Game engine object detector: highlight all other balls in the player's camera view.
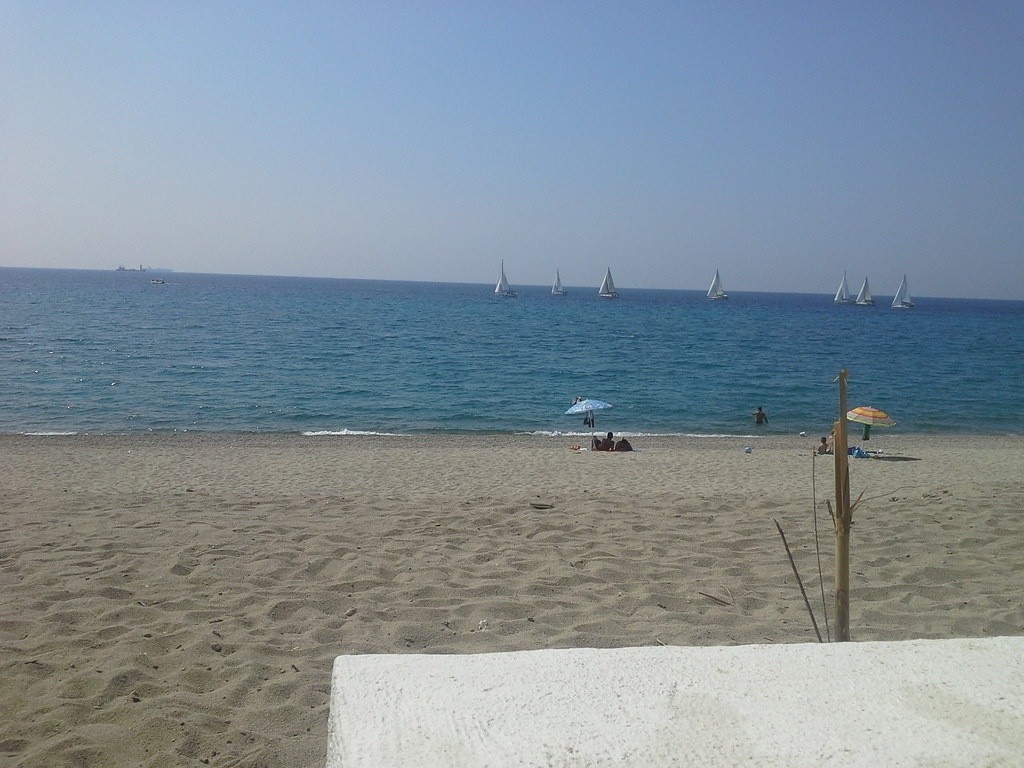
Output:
[745,447,752,453]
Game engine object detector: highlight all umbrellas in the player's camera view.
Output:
[847,406,897,451]
[565,398,612,450]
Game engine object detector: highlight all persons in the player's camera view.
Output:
[592,432,615,451]
[828,429,835,454]
[817,436,828,455]
[753,407,769,424]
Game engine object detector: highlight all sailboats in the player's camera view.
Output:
[854,276,876,305]
[833,271,855,302]
[706,268,727,299]
[552,268,565,295]
[494,259,517,297]
[598,267,618,298]
[891,274,915,309]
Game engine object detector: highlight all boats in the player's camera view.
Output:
[150,279,167,284]
